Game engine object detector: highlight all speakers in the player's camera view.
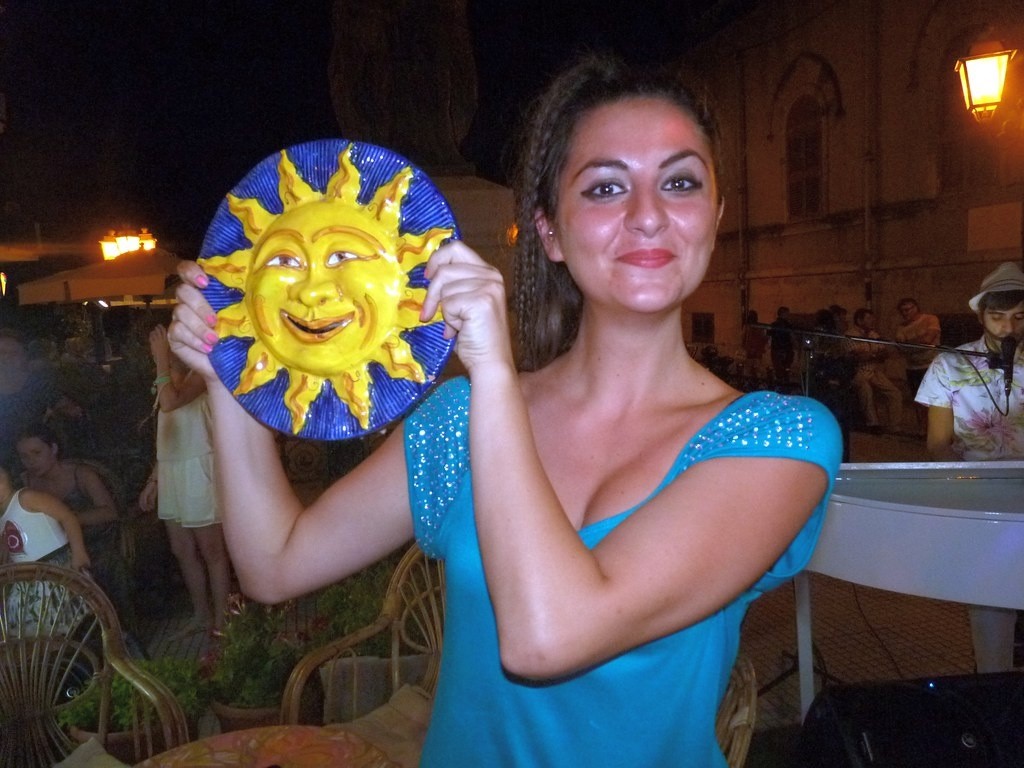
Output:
[797,669,1024,768]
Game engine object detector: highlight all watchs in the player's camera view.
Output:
[145,476,158,484]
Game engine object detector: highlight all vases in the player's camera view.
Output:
[207,684,317,733]
[320,652,429,722]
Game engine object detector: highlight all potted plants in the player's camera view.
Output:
[55,657,207,765]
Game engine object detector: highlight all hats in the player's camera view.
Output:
[968,261,1024,313]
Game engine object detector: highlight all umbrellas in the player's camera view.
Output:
[16,242,192,320]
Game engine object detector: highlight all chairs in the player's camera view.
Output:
[0,560,189,768]
[59,459,152,661]
[279,542,445,725]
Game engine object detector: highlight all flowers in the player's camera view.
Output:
[309,557,441,658]
[201,592,318,708]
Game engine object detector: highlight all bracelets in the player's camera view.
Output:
[150,367,174,395]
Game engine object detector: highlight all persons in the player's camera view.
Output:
[0,461,93,642]
[136,303,234,641]
[895,295,942,441]
[163,48,847,768]
[843,305,909,438]
[61,315,114,363]
[913,256,1024,678]
[745,303,848,398]
[11,423,136,633]
[1,326,86,494]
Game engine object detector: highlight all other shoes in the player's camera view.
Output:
[167,610,212,639]
[889,429,905,436]
[870,424,884,434]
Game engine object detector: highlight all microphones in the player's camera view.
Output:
[999,336,1017,398]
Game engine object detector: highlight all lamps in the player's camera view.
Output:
[954,24,1024,149]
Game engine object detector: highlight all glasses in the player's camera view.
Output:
[900,304,916,315]
